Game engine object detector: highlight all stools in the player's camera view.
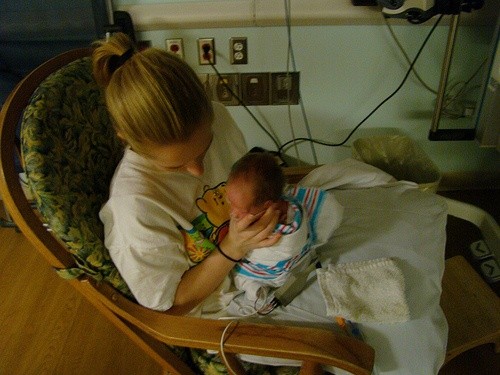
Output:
[439,255,500,370]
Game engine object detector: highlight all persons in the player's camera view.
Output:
[223,153,311,300]
[92,31,336,375]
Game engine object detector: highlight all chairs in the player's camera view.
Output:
[0,47,379,375]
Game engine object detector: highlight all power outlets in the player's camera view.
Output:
[165,39,185,60]
[197,38,216,65]
[196,71,301,107]
[229,37,247,65]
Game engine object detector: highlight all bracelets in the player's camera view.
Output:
[218,241,248,265]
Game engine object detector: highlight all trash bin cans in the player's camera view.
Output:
[353,135,442,193]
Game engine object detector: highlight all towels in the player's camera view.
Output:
[316,256,410,324]
